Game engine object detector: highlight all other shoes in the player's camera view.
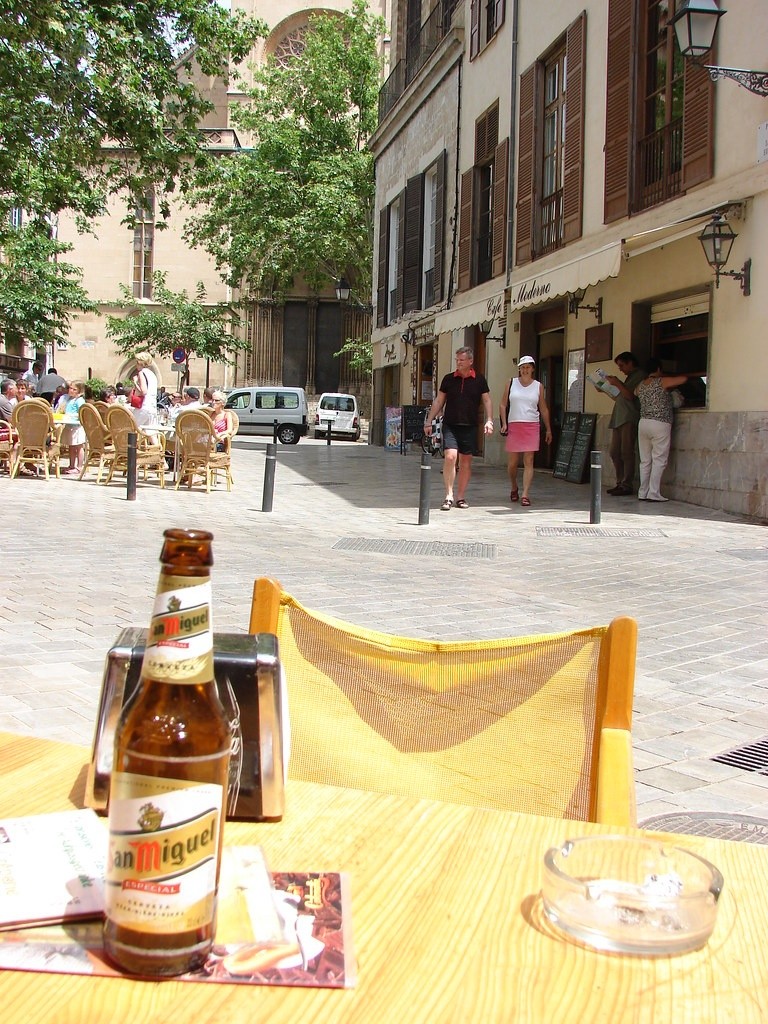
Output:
[639,495,669,503]
[24,462,42,472]
[61,465,76,474]
[66,467,82,473]
[20,468,35,476]
[606,486,633,495]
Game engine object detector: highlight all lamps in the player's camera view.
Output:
[333,277,373,317]
[399,329,415,347]
[477,319,506,349]
[568,287,602,324]
[665,0,768,98]
[697,211,751,297]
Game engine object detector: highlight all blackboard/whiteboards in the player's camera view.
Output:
[552,411,581,480]
[565,412,598,483]
[400,405,426,443]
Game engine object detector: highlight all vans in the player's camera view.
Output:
[314,392,364,441]
[222,385,310,445]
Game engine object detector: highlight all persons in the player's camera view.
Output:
[595,352,647,495]
[129,352,169,469]
[126,386,233,482]
[0,359,136,476]
[499,356,552,506]
[634,357,690,501]
[424,346,494,510]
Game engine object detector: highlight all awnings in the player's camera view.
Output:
[432,289,512,335]
[510,212,719,314]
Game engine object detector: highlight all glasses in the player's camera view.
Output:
[211,399,220,402]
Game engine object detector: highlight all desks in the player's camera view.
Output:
[0,728,768,1024]
[140,424,218,483]
[53,419,81,425]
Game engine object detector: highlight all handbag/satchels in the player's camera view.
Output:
[499,378,514,436]
[130,395,144,408]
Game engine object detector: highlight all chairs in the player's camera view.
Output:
[247,577,636,829]
[256,395,263,408]
[344,405,352,410]
[328,404,333,410]
[275,396,285,408]
[0,397,238,493]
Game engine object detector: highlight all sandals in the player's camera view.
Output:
[456,499,468,508]
[441,500,453,510]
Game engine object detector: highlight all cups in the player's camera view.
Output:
[148,409,174,426]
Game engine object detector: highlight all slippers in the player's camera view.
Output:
[521,495,531,505]
[510,486,519,502]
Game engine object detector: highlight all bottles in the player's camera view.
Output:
[102,528,232,977]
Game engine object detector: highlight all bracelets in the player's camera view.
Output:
[487,420,493,425]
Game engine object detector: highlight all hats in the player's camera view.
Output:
[184,388,200,399]
[518,356,535,366]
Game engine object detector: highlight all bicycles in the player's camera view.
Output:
[419,403,445,458]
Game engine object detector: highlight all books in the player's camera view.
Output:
[0,809,359,990]
